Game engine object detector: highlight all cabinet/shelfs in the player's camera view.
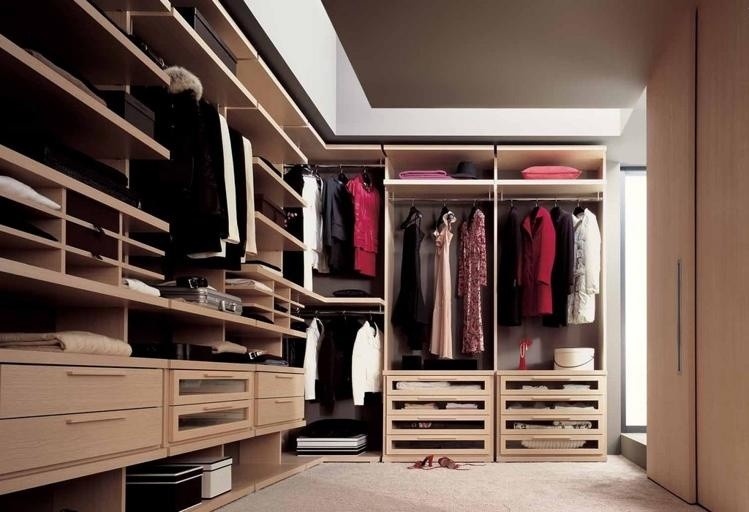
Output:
[1,2,383,512]
[384,144,607,463]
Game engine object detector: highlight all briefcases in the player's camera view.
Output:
[154,286,242,315]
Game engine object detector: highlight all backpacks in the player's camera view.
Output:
[553,347,595,370]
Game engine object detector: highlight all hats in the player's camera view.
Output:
[451,161,480,179]
